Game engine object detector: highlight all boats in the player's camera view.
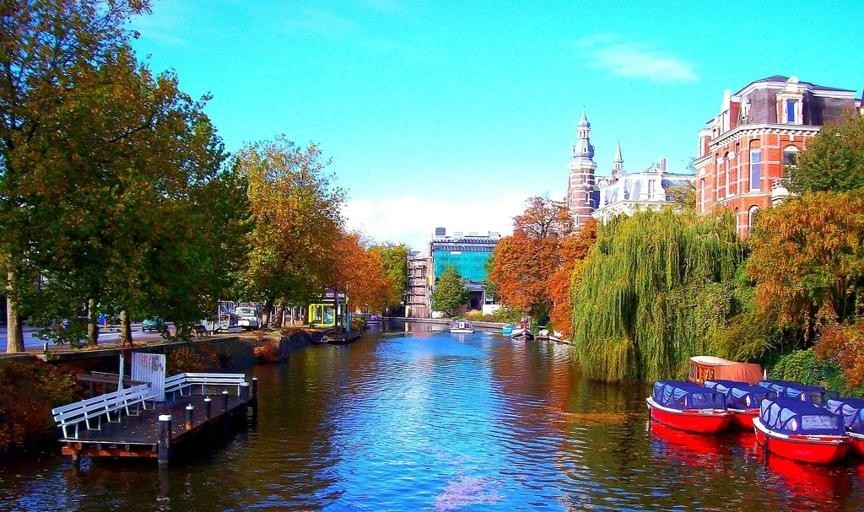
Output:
[500,321,533,339]
[646,354,864,465]
[448,319,475,333]
[306,283,362,345]
[365,314,383,324]
[645,417,863,512]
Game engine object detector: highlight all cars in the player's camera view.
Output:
[141,316,168,332]
[60,317,99,338]
